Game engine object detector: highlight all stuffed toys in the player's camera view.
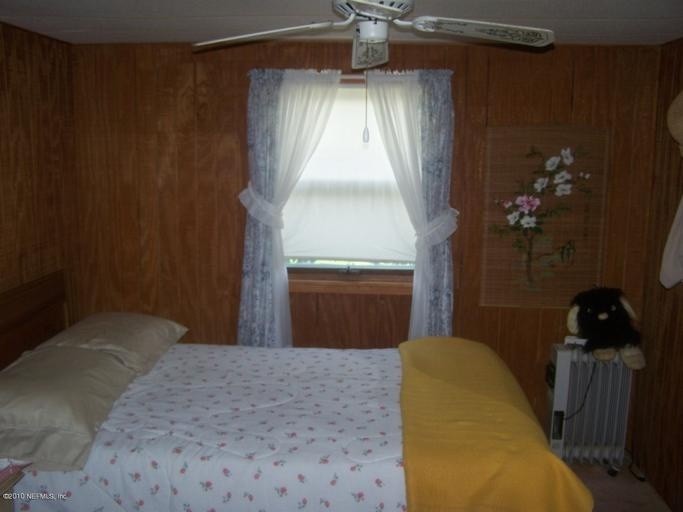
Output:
[565,286,647,370]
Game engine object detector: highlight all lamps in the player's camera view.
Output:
[357,21,388,144]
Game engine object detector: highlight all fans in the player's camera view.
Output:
[190,1,556,143]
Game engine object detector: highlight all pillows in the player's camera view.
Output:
[32,310,189,376]
[1,346,138,472]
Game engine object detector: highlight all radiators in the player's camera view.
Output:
[540,342,634,476]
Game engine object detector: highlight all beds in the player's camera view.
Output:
[0,266,593,511]
